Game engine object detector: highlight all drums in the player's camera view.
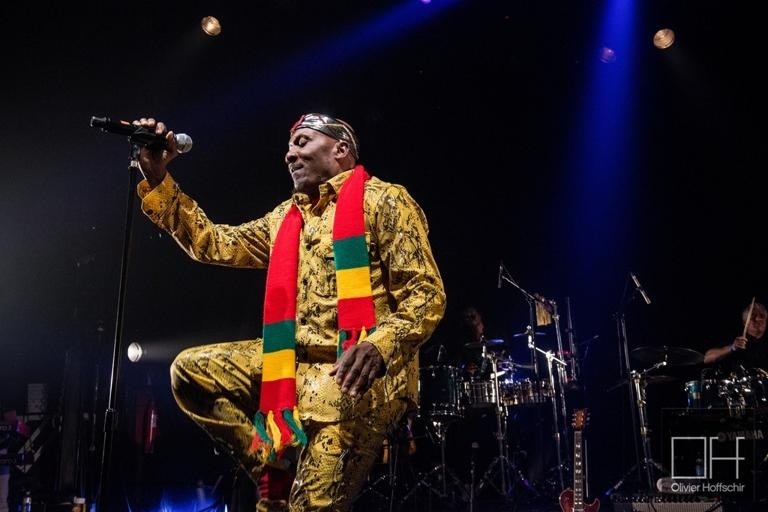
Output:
[419,366,465,421]
[500,382,524,405]
[683,380,718,410]
[546,350,580,384]
[522,378,552,404]
[470,380,497,408]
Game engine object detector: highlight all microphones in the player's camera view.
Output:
[628,270,652,305]
[88,113,195,156]
[495,258,509,288]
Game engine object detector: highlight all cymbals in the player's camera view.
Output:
[514,332,545,337]
[630,347,705,367]
[465,339,504,348]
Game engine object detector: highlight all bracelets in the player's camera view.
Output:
[729,343,735,353]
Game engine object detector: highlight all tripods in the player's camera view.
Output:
[346,302,718,511]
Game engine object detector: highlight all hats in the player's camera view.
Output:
[289,111,361,162]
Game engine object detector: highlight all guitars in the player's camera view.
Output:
[559,408,600,512]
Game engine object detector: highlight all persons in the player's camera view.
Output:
[456,305,499,373]
[132,112,448,512]
[702,302,766,371]
